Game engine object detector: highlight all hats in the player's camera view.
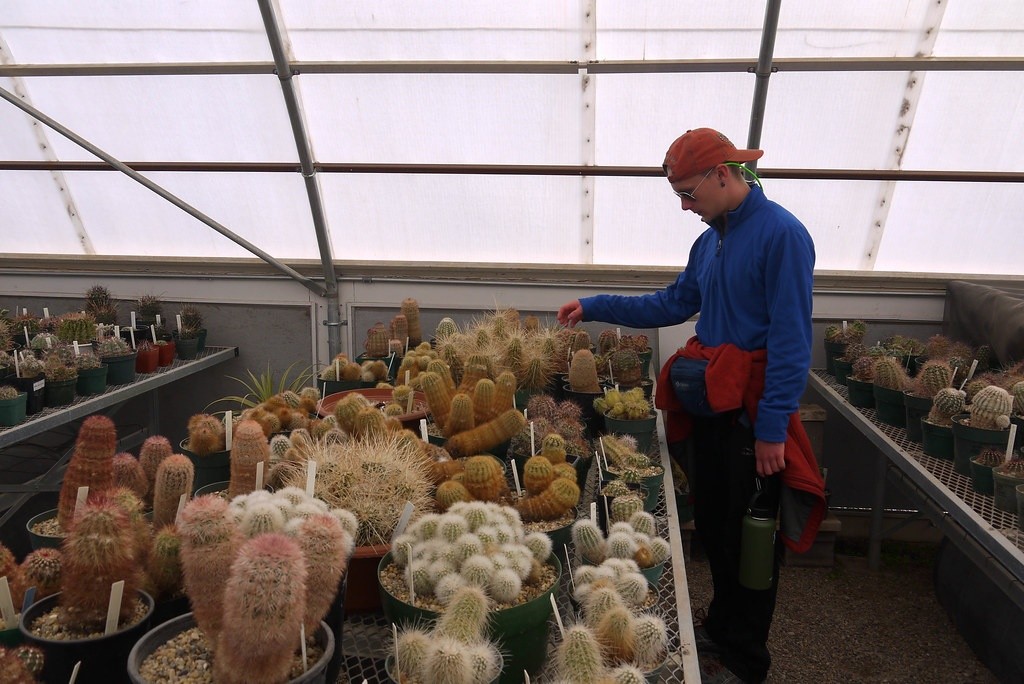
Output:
[661,127,764,183]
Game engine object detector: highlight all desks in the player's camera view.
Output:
[809,365,1024,610]
[0,346,239,525]
[0,361,706,684]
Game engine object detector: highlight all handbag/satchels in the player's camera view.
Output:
[668,353,713,417]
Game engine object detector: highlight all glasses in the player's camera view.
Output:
[673,169,714,203]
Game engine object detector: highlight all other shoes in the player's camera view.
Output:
[702,659,744,683]
[696,610,726,654]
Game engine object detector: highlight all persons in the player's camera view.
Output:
[557,132,826,684]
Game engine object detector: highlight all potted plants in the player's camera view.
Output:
[0,284,1024,684]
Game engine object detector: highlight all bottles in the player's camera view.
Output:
[738,497,777,590]
